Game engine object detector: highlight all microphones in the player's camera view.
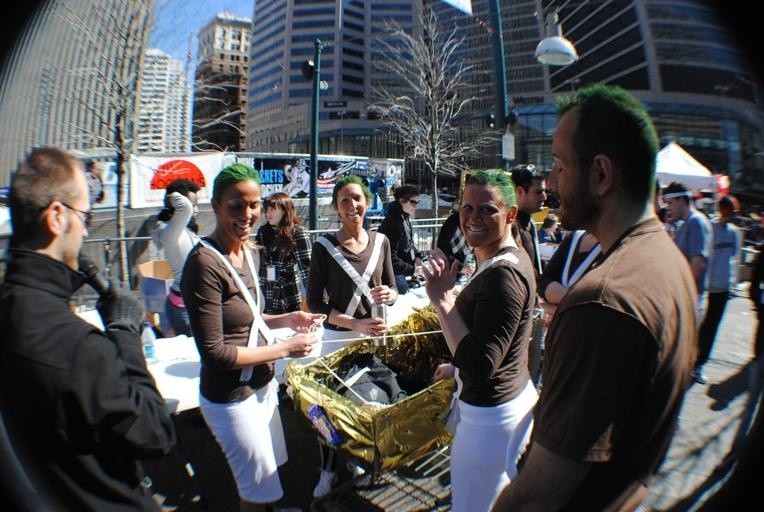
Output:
[77,254,110,297]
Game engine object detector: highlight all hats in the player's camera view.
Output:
[542,213,558,229]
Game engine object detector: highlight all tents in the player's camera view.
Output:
[655,140,714,191]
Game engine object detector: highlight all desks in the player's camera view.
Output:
[144,282,455,448]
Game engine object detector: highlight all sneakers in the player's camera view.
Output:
[693,367,708,385]
[313,470,335,498]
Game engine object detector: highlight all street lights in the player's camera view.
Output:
[484,0,580,175]
[301,36,327,236]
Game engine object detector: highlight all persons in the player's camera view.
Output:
[509,162,547,385]
[491,85,700,512]
[376,186,422,294]
[692,196,739,384]
[88,159,104,204]
[305,175,399,497]
[0,145,177,512]
[437,212,474,282]
[535,229,605,325]
[537,213,562,243]
[179,162,328,512]
[662,182,713,317]
[419,168,539,512]
[155,178,201,338]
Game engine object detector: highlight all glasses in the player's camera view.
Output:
[64,203,95,228]
[408,198,421,206]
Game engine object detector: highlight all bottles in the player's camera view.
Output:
[141,322,158,364]
[372,303,386,346]
